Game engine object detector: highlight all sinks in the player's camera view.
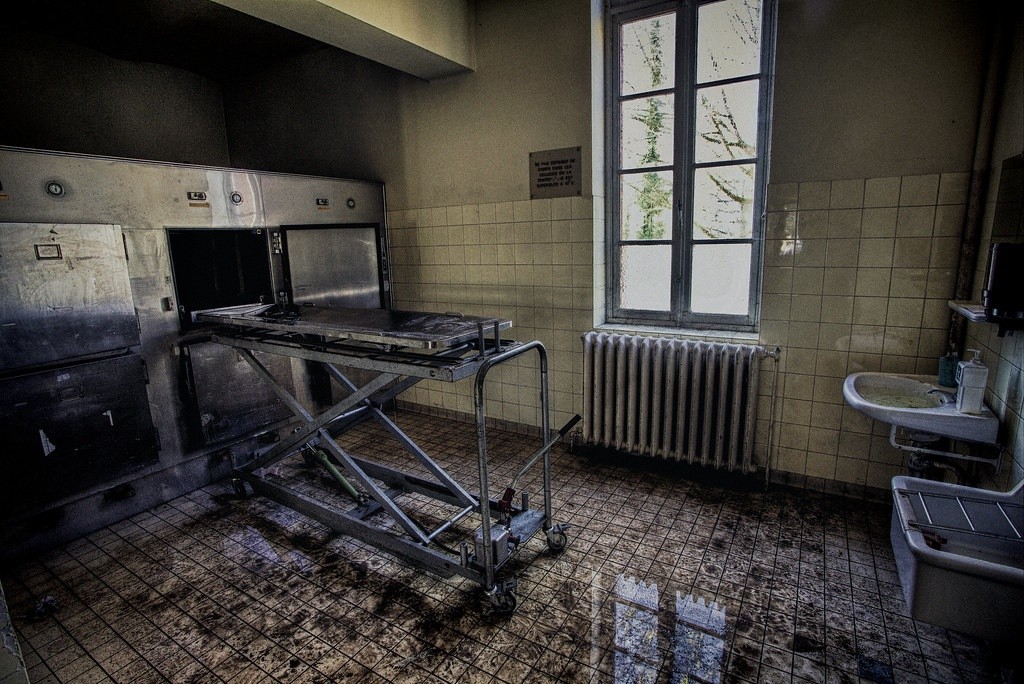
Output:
[842,371,1000,445]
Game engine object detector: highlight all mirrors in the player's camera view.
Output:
[983,153,1024,312]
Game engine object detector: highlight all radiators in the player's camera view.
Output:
[581,331,767,477]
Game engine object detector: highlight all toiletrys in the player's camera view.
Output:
[939,345,959,388]
[955,348,989,416]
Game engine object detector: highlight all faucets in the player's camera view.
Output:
[926,388,955,404]
